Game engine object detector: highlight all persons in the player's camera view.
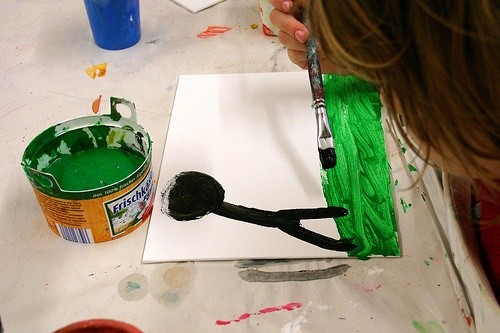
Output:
[269,0,499,299]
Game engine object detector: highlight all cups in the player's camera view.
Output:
[52,318,144,333]
[83,0,141,51]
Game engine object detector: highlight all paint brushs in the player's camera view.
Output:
[295,1,339,169]
